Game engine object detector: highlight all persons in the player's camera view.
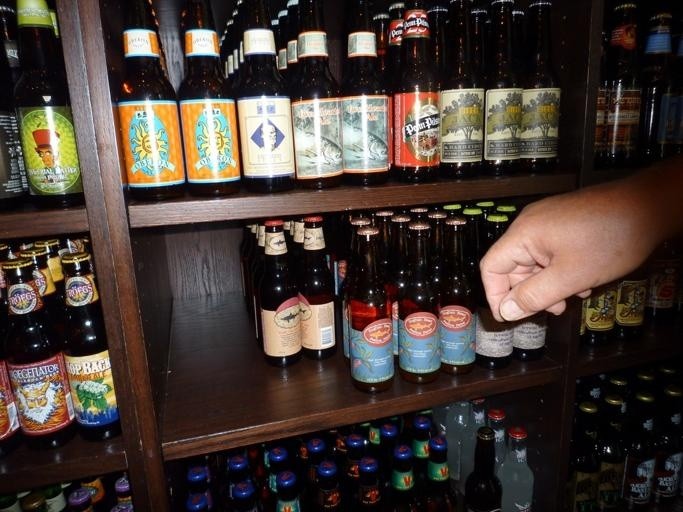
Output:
[260,125,279,152]
[480,148,683,323]
[32,129,66,184]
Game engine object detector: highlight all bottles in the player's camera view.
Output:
[1,469,132,510]
[179,380,535,510]
[1,1,86,211]
[590,3,683,173]
[565,363,681,509]
[1,230,126,454]
[579,233,681,348]
[239,194,550,399]
[99,2,588,206]
